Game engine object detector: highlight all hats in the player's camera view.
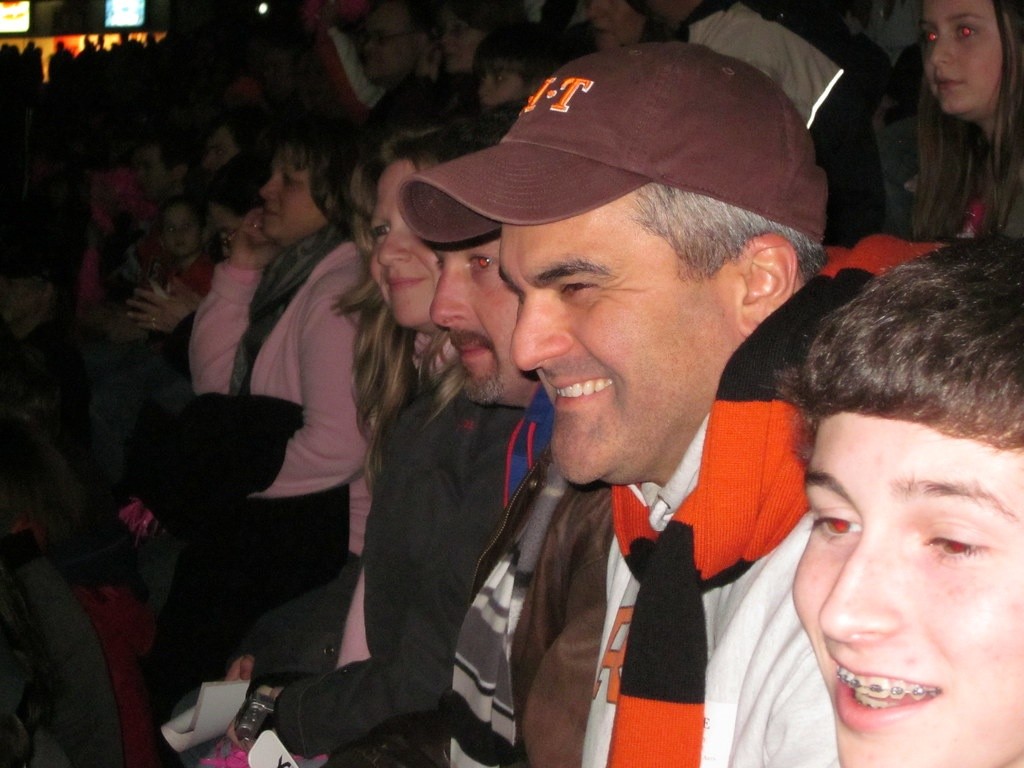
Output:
[395,41,828,242]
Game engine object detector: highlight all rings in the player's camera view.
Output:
[153,322,155,329]
[152,316,155,322]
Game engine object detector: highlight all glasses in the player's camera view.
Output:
[357,27,426,46]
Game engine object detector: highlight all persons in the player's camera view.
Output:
[793,238,1024,768]
[0,100,554,768]
[0,32,156,87]
[262,0,1024,248]
[396,41,877,768]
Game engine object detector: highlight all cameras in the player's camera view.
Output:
[235,699,276,740]
